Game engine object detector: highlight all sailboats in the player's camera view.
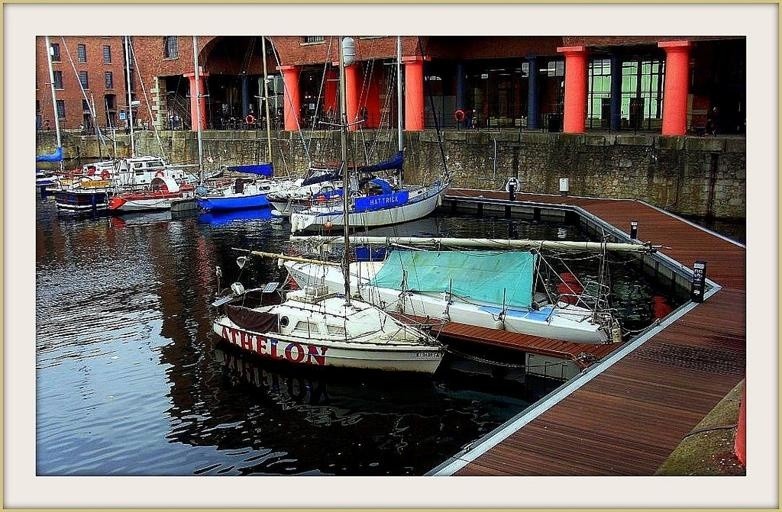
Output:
[207,43,447,373]
[229,232,667,343]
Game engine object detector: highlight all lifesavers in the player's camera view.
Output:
[455,109,465,122]
[506,177,520,192]
[246,114,255,124]
[155,170,164,178]
